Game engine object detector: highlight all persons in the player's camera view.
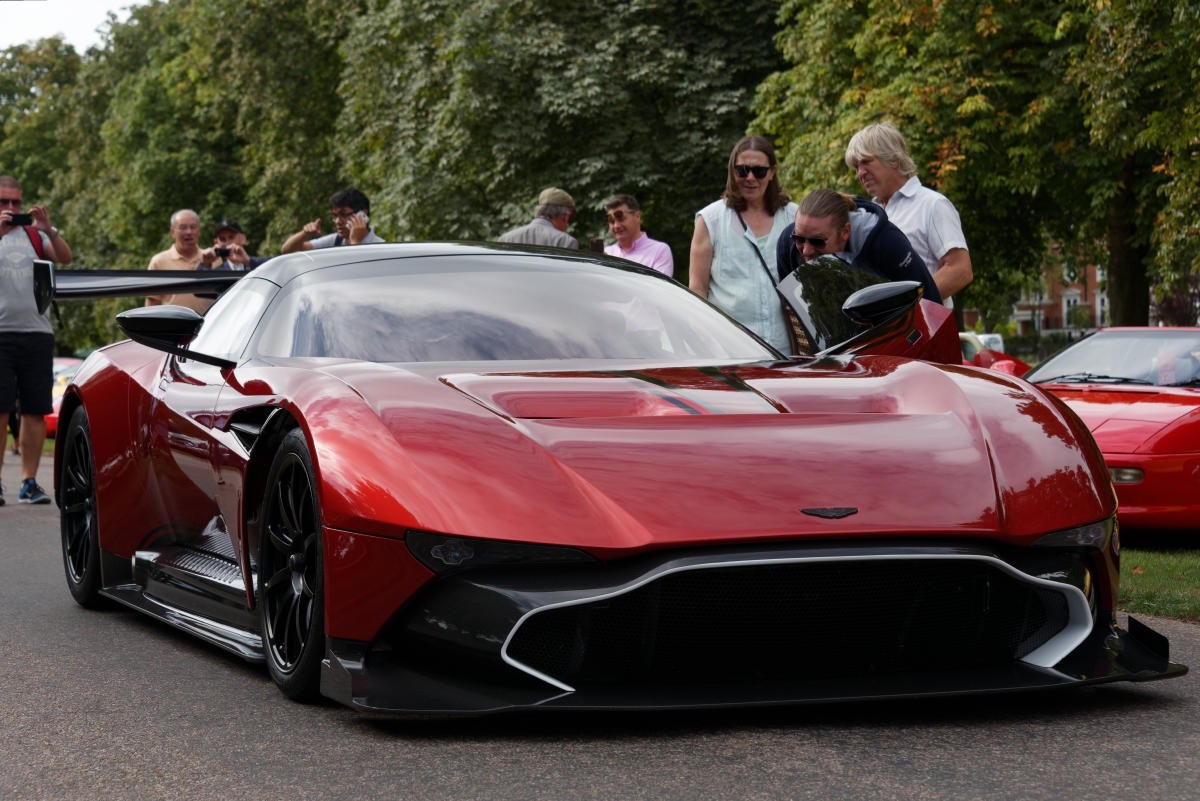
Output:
[144,209,223,316]
[281,187,386,254]
[206,433,226,485]
[7,409,20,455]
[497,187,578,250]
[776,189,944,352]
[0,175,73,507]
[599,194,673,278]
[192,219,272,300]
[844,121,973,308]
[688,136,799,359]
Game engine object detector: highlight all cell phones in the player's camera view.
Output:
[345,211,370,231]
[214,248,230,258]
[6,213,33,227]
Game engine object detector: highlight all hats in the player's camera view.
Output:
[214,220,242,237]
[539,188,576,215]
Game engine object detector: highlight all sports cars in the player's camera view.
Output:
[28,237,1191,722]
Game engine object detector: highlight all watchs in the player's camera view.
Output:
[49,226,58,239]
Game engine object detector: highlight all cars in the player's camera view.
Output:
[990,325,1200,535]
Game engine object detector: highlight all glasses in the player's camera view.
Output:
[0,198,21,207]
[330,207,361,222]
[735,163,772,180]
[789,229,840,249]
[604,208,637,224]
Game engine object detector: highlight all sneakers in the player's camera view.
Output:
[0,485,6,506]
[18,478,51,504]
